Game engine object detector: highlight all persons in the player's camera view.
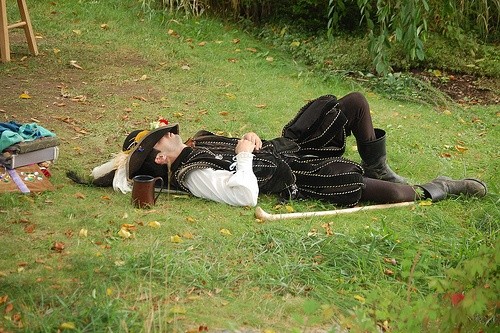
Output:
[93,92,489,205]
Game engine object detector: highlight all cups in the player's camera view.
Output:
[131,175,164,208]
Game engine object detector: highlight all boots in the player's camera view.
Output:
[416,176,488,202]
[356,127,407,184]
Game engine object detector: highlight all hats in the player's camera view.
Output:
[66,119,179,193]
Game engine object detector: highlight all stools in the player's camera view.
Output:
[0,0,39,62]
